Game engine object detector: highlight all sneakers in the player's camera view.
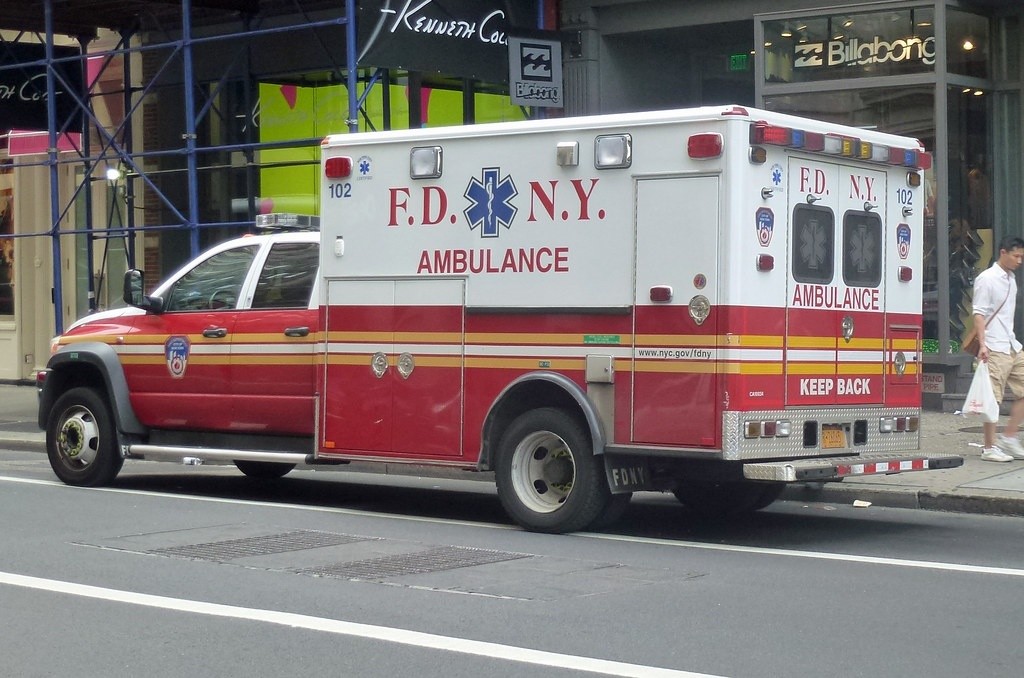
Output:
[997,436,1024,459]
[981,445,1013,462]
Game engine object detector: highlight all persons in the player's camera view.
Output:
[972,235,1024,462]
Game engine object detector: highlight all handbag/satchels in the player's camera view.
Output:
[962,360,999,423]
[960,328,980,357]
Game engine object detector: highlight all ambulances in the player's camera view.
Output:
[33,102,964,536]
